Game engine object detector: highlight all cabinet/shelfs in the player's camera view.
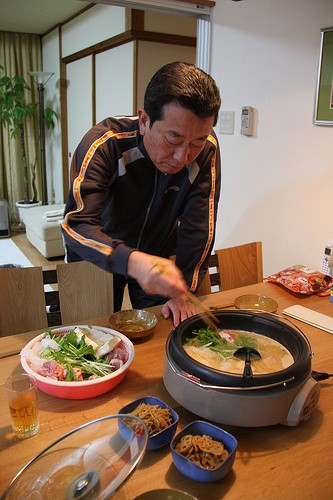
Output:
[37,5,196,205]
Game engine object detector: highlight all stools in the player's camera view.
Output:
[23,203,66,259]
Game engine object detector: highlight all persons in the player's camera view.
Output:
[61,60,222,328]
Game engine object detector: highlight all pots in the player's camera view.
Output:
[162,309,322,427]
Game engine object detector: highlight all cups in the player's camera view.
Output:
[6,373,40,439]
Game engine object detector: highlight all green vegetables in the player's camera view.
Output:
[40,328,116,382]
[188,326,262,362]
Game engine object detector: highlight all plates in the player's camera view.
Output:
[268,263,333,295]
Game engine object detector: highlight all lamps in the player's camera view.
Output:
[28,70,54,205]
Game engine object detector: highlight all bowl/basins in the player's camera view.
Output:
[108,308,158,339]
[133,488,197,500]
[20,324,135,399]
[170,420,238,484]
[118,396,179,451]
[234,294,278,313]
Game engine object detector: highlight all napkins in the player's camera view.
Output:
[282,305,333,335]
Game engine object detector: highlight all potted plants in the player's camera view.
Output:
[0,62,59,226]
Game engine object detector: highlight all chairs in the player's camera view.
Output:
[0,261,115,338]
[169,241,264,296]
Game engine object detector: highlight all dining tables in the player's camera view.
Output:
[0,281,333,500]
